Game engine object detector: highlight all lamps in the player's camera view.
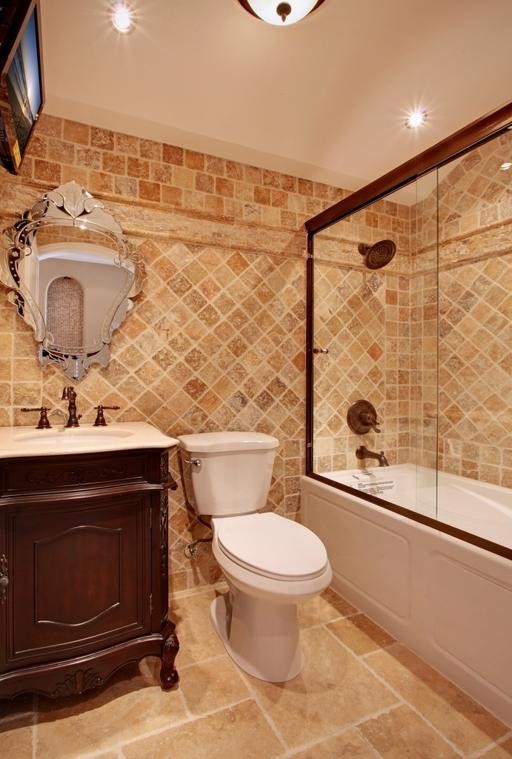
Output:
[235,0,324,25]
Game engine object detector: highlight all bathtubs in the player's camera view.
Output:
[319,461,512,550]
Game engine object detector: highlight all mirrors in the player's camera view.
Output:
[0,178,148,378]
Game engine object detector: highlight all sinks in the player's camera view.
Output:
[13,426,138,454]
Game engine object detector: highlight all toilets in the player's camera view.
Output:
[176,431,333,683]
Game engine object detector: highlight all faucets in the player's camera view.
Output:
[61,385,82,428]
[356,444,389,467]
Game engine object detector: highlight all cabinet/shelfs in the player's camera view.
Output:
[1,451,179,707]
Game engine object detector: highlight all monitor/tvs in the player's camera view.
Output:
[0,1,46,176]
